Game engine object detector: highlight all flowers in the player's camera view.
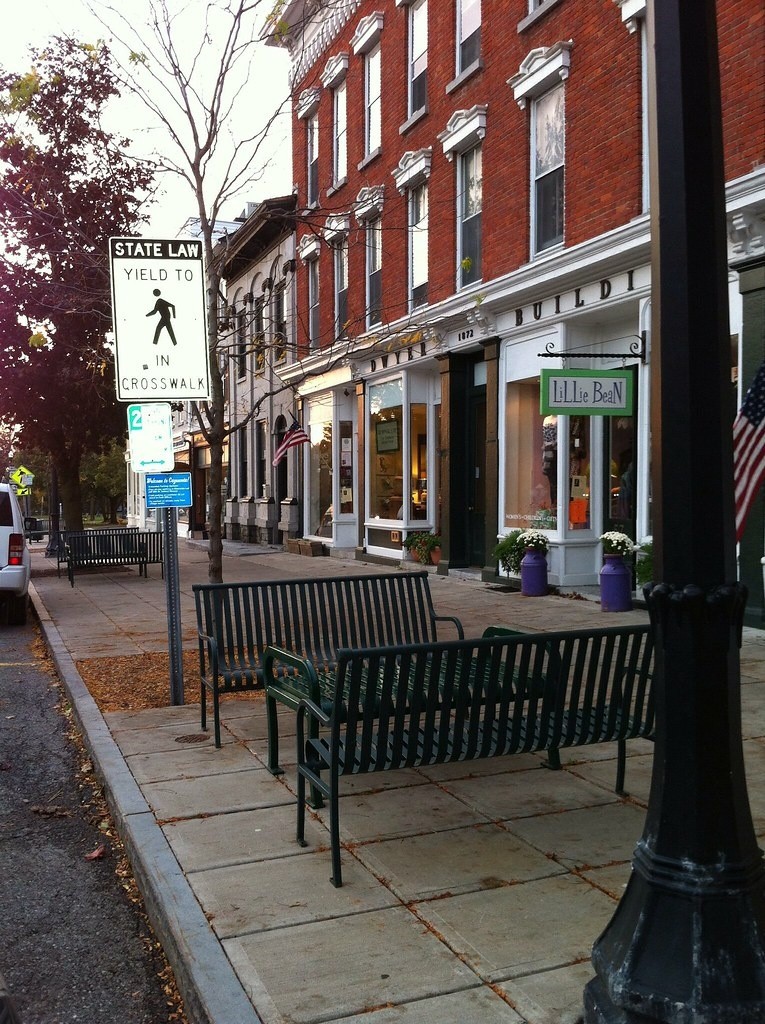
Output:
[634,535,654,591]
[599,531,634,555]
[516,531,551,555]
[399,530,441,565]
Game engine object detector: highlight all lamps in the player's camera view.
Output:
[416,478,427,502]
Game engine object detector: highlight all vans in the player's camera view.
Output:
[0,482,37,626]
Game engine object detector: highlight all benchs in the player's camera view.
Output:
[68,542,148,582]
[191,570,464,749]
[67,531,165,588]
[262,624,561,811]
[26,519,65,544]
[57,527,143,579]
[295,623,656,889]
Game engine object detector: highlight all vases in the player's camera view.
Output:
[604,554,621,559]
[524,547,538,553]
[410,546,421,562]
[298,540,322,557]
[428,546,441,565]
[286,537,309,554]
